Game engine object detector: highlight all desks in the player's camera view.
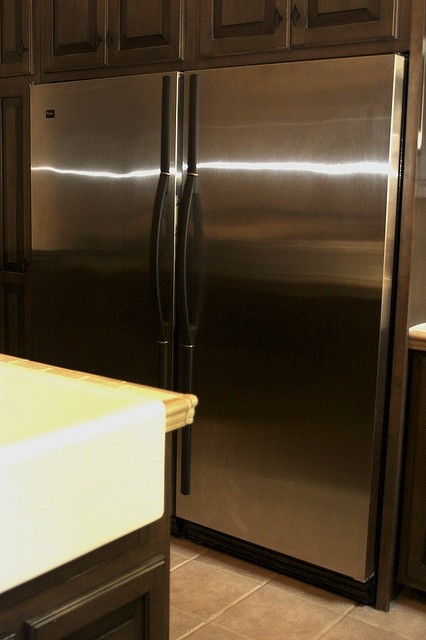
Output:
[0,354,198,640]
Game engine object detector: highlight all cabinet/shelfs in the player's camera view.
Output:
[0,0,37,77]
[37,0,193,72]
[0,79,29,275]
[1,273,27,360]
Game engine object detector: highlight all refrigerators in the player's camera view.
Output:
[30,51,410,582]
